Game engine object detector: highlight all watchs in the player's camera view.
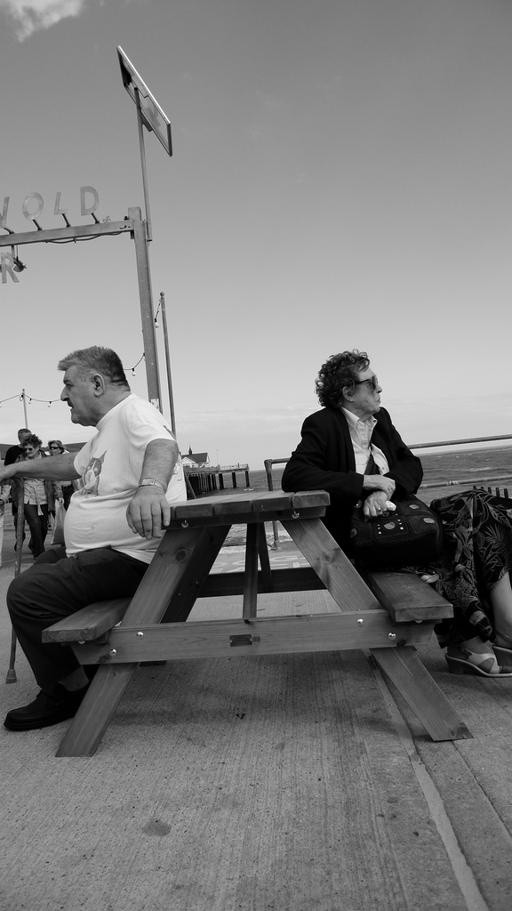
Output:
[137,478,163,488]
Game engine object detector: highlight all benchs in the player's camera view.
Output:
[42,590,164,760]
[366,563,475,743]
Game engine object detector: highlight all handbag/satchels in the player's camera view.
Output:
[341,499,439,569]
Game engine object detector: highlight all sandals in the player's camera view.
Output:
[446,630,512,677]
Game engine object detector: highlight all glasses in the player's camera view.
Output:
[357,376,377,390]
[50,447,58,449]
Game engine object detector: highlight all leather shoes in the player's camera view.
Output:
[2,690,84,731]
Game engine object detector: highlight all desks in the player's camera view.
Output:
[58,487,477,754]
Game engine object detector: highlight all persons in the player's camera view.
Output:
[282,351,512,677]
[0,345,186,731]
[0,428,75,558]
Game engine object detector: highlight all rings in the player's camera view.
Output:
[376,508,382,512]
